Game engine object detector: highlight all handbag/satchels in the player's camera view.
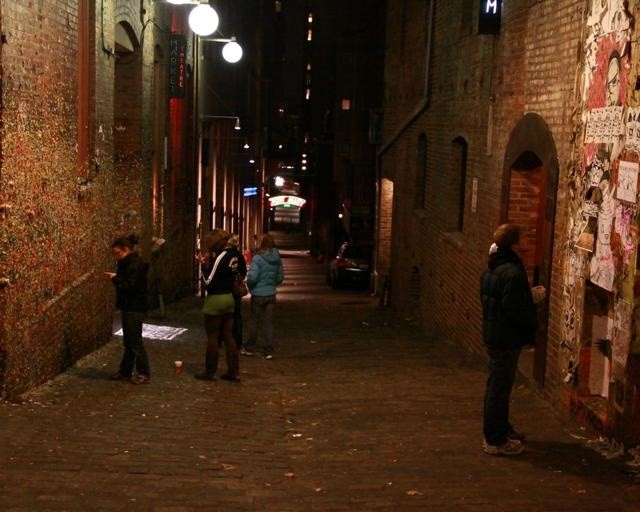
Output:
[231,277,248,299]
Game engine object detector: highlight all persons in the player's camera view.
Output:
[103,234,154,384]
[479,222,546,456]
[196,228,284,383]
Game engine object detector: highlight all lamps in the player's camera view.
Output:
[206,114,241,131]
[200,35,245,63]
[154,0,219,36]
[224,134,251,150]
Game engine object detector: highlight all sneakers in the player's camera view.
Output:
[220,372,241,382]
[260,353,274,359]
[194,369,217,381]
[483,438,522,457]
[105,372,133,382]
[130,374,154,384]
[240,348,256,357]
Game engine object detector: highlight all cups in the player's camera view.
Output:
[174,360,182,374]
